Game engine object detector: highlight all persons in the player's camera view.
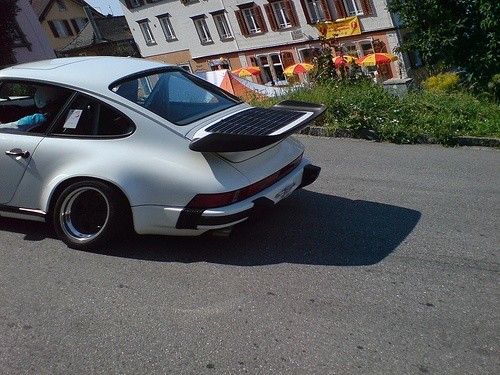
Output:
[377,65,383,82]
[0,85,59,130]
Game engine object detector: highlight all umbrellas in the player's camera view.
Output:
[283,63,315,77]
[355,52,398,81]
[327,55,358,68]
[231,66,262,79]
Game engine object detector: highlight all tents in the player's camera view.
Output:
[194,69,297,106]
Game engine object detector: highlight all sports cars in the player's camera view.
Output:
[0,55,328,252]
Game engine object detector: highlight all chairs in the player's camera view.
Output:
[64,102,100,139]
